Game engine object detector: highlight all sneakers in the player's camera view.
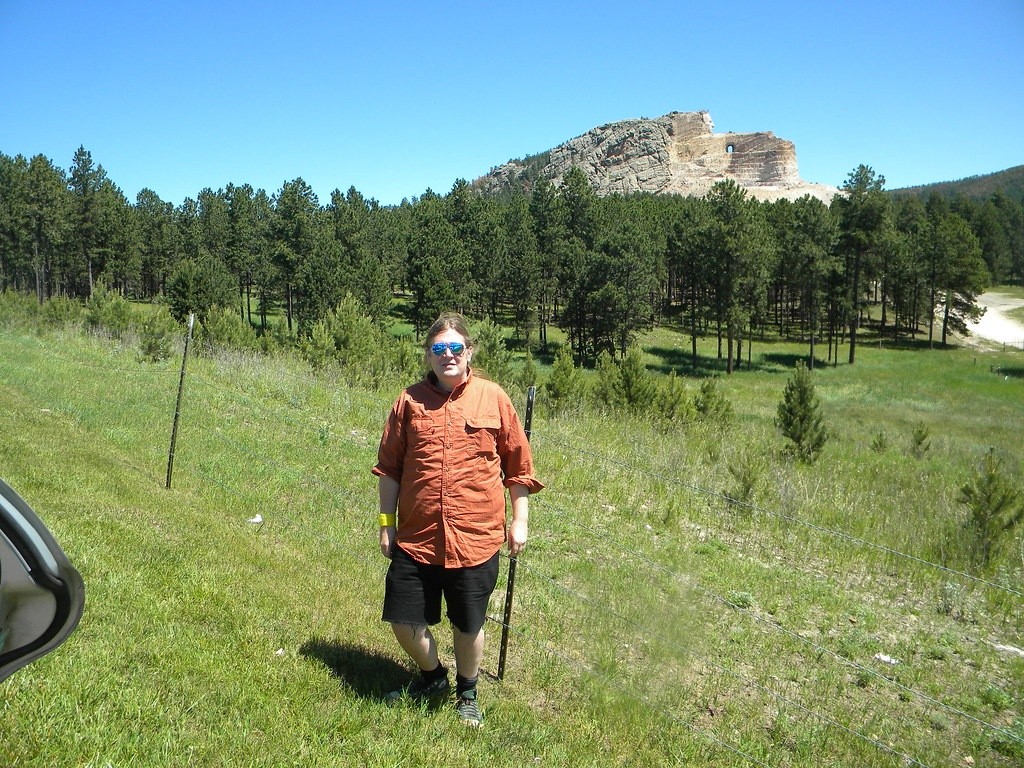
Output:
[389,671,449,702]
[450,683,480,728]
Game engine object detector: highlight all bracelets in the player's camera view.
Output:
[377,514,396,528]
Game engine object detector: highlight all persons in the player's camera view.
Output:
[369,313,546,728]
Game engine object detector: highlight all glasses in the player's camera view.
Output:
[428,342,468,356]
